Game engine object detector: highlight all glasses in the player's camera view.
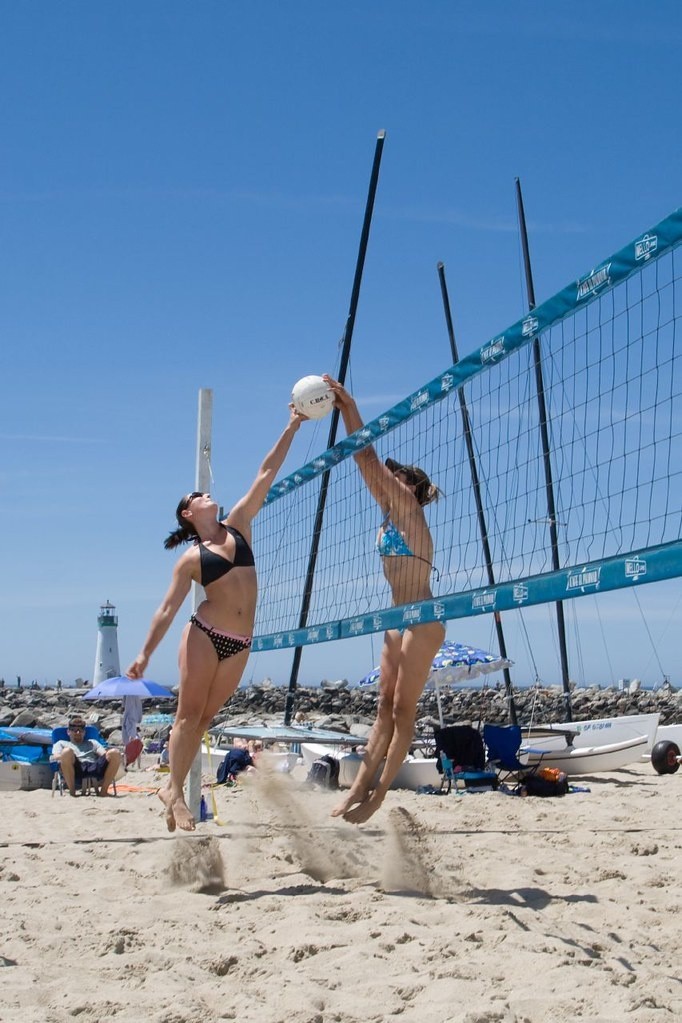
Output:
[68,725,86,732]
[184,492,202,510]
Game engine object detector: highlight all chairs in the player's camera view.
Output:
[51,726,117,797]
[433,725,498,794]
[484,725,552,796]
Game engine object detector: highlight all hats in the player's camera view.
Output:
[386,457,420,496]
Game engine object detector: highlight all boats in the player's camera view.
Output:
[291,710,661,789]
[638,724,682,762]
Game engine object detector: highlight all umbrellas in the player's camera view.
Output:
[142,712,174,740]
[81,677,177,701]
[358,639,515,728]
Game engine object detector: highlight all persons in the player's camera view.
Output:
[126,402,309,831]
[129,726,142,769]
[141,734,152,747]
[51,718,122,797]
[322,372,445,825]
[161,741,170,764]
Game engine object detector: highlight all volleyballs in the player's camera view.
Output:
[292,377,334,418]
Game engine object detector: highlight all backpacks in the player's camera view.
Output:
[306,755,339,790]
